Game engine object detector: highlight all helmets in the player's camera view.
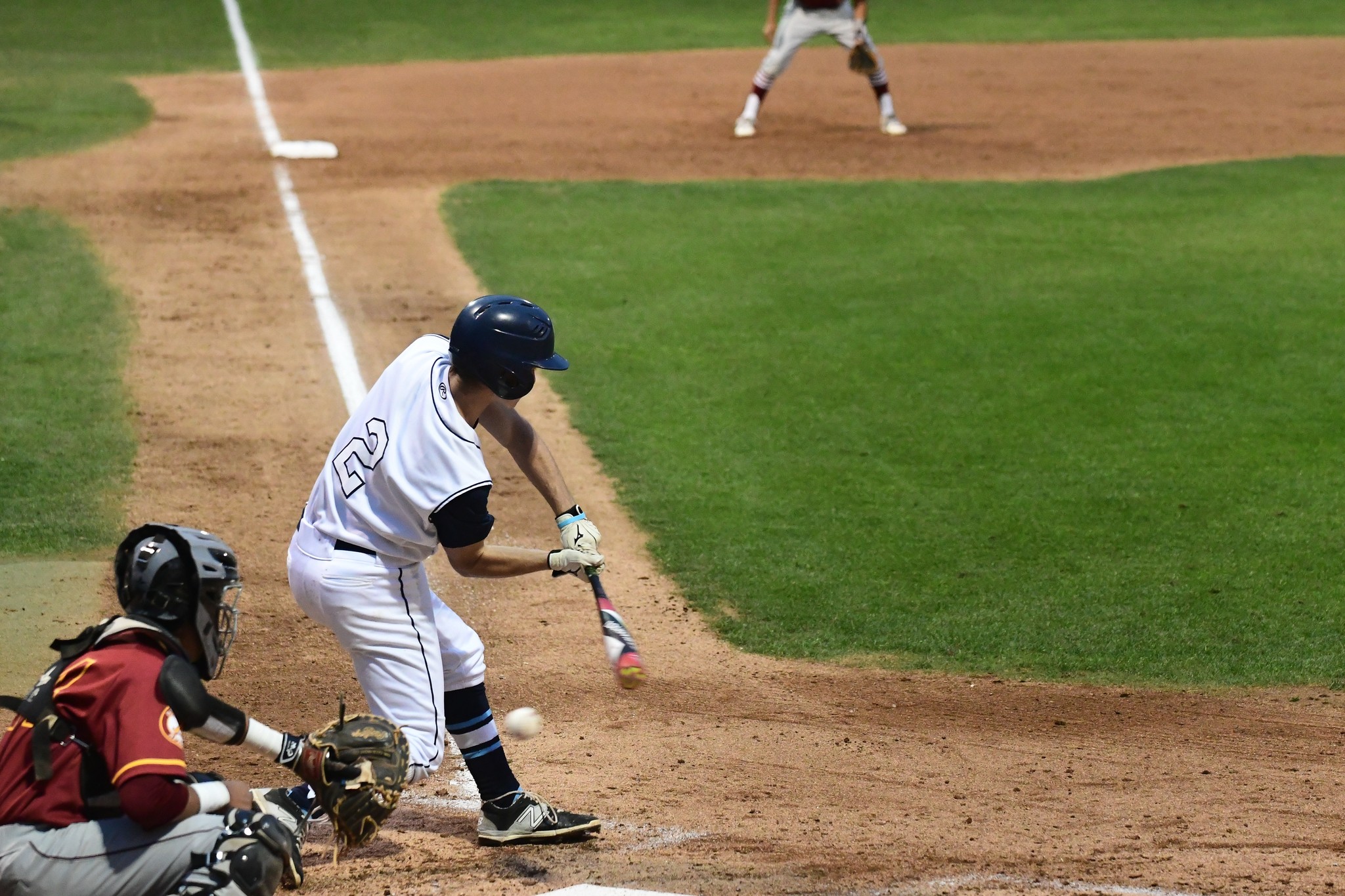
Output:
[449,296,569,400]
[113,521,241,681]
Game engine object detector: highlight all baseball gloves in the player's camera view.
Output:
[301,713,407,848]
[849,39,878,75]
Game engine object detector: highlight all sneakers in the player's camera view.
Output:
[733,115,755,138]
[477,787,602,845]
[248,788,329,854]
[881,118,906,137]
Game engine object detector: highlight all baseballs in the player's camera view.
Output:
[500,708,547,739]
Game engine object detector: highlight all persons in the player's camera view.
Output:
[733,0,908,137]
[286,294,606,847]
[0,522,362,896]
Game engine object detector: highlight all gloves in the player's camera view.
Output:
[547,545,606,583]
[555,503,601,551]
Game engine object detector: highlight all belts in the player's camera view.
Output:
[296,508,376,556]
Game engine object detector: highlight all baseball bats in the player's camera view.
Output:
[585,563,647,690]
